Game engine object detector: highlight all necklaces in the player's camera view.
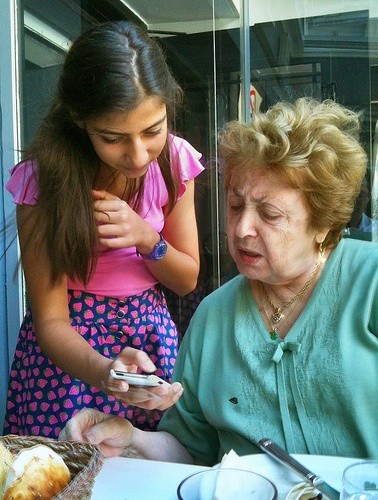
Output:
[257,248,327,339]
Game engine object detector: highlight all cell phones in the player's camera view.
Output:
[110,369,171,388]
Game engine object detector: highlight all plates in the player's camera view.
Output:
[199,452,377,499]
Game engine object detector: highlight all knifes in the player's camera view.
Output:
[258,437,340,500]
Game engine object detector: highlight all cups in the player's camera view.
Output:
[176,468,278,500]
[340,460,378,500]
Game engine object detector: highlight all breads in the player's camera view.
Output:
[0,441,71,500]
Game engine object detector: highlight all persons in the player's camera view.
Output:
[58,98,378,466]
[3,22,204,442]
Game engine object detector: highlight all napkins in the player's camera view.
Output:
[213,449,314,500]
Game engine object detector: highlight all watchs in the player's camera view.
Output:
[136,232,168,261]
